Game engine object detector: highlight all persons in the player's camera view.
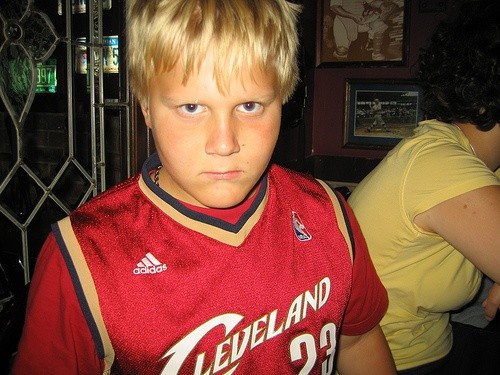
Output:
[15,0,398,375]
[330,0,392,61]
[347,0,500,375]
[358,97,417,132]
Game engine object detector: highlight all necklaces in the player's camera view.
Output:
[153,162,163,187]
[451,123,478,158]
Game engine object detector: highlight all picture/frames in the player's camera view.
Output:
[343,78,426,149]
[317,0,410,69]
